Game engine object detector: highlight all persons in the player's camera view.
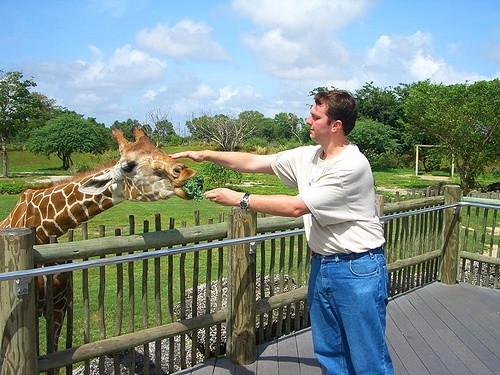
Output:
[169,90,398,375]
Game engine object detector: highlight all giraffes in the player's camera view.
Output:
[0,124,199,375]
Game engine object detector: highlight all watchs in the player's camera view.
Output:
[239,194,250,210]
